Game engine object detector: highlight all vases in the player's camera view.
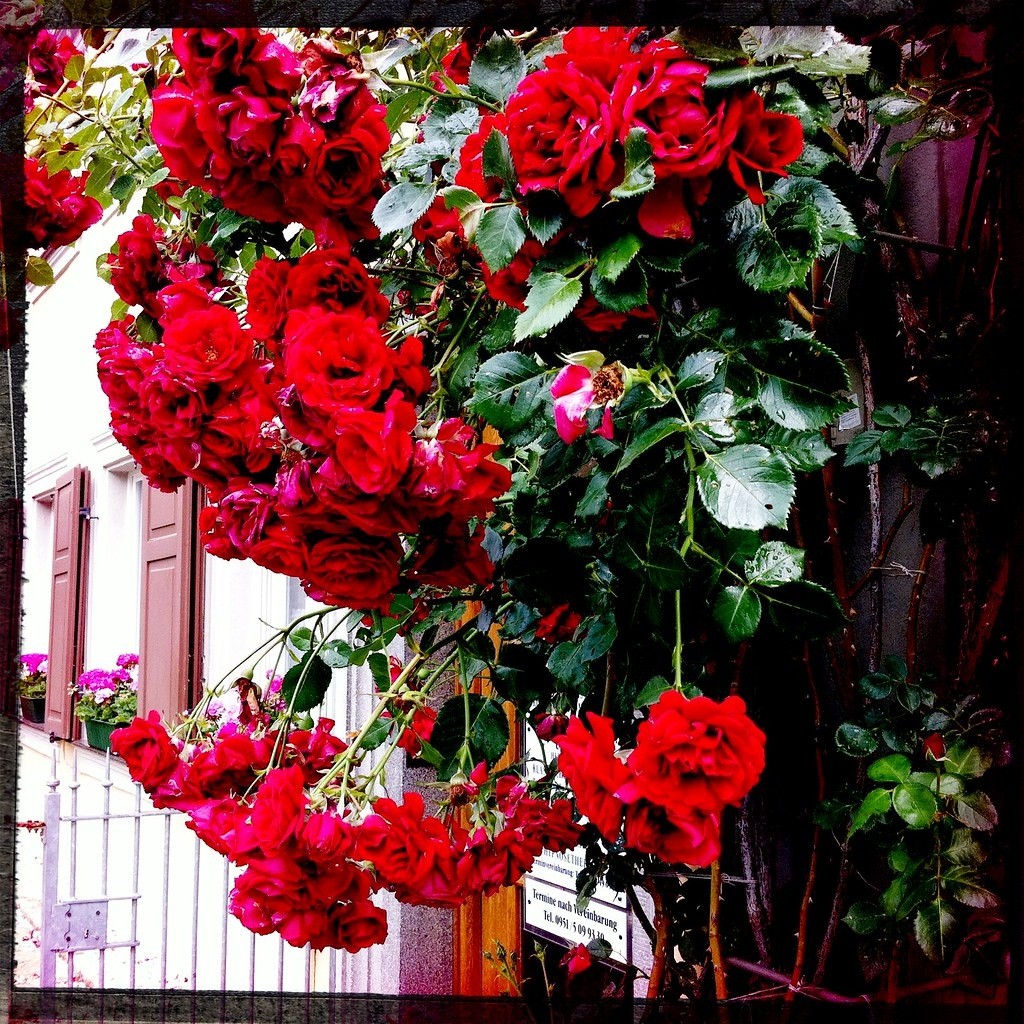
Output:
[83,720,130,752]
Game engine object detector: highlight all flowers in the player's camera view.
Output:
[68,653,140,719]
[21,654,50,697]
[21,697,46,722]
